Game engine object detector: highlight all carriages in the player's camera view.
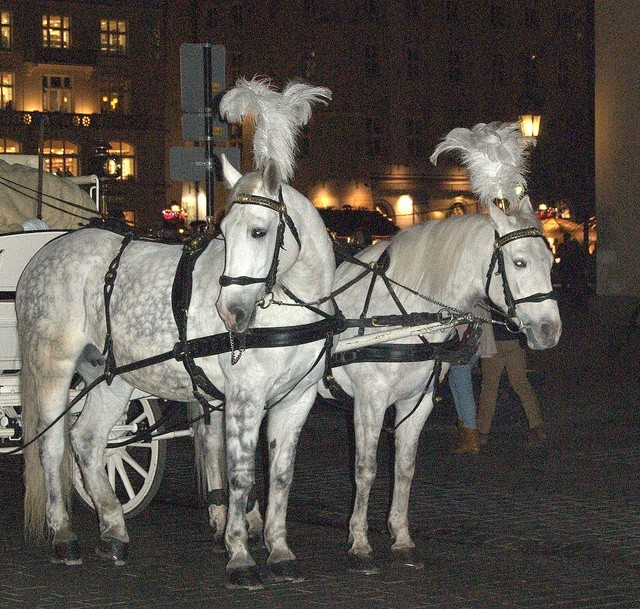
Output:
[1,73,562,590]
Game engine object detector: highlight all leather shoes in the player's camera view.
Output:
[452,432,480,457]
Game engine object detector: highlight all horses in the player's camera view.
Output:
[187,120,564,579]
[14,74,341,594]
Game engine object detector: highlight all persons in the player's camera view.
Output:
[555,230,585,289]
[475,295,550,450]
[447,354,484,460]
